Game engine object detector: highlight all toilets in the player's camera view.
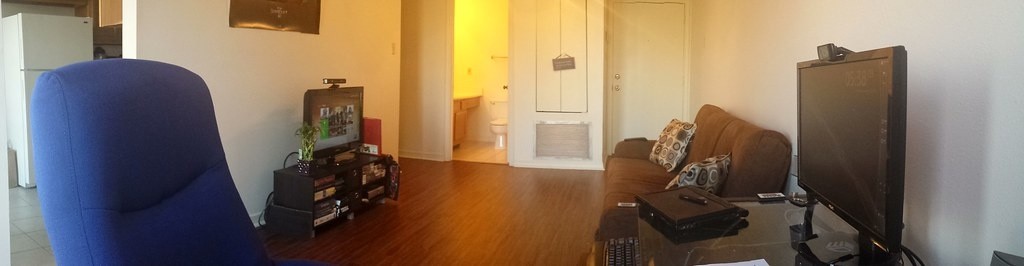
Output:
[489,99,508,150]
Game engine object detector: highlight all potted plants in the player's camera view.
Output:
[297,122,319,177]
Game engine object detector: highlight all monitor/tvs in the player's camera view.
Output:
[795,46,907,266]
[304,87,363,168]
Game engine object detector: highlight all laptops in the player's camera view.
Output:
[633,184,749,229]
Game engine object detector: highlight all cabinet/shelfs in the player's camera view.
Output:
[266,152,401,240]
[453,111,467,148]
[98,0,122,29]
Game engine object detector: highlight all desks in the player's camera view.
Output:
[638,193,904,266]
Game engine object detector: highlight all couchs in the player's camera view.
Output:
[594,104,792,239]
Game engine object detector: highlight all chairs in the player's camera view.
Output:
[32,57,279,265]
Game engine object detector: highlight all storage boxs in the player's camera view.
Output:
[363,116,381,156]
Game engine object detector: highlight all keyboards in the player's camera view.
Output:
[602,236,640,266]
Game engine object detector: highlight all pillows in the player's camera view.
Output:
[664,152,733,195]
[649,117,697,173]
